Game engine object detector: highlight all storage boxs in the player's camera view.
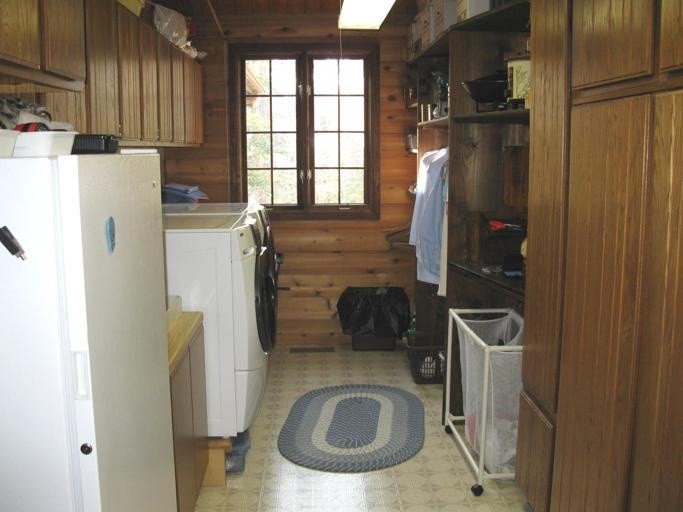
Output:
[167,295,182,336]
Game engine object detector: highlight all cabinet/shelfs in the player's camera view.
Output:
[514,2,682,510]
[0,0,205,148]
[402,0,530,321]
[169,324,210,512]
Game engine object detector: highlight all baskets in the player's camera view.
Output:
[406,341,445,386]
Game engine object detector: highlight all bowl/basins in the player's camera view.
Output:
[460,79,507,103]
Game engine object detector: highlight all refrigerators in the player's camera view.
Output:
[0,150,178,512]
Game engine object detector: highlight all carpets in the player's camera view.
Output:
[277,384,425,474]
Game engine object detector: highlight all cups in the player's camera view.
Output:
[427,103,436,121]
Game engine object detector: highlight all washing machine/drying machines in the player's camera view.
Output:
[163,201,282,439]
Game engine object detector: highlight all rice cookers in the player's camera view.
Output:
[505,54,530,109]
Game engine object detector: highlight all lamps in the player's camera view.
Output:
[338,0,396,31]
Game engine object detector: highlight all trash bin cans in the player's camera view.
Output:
[444,307,525,496]
[352,287,395,350]
